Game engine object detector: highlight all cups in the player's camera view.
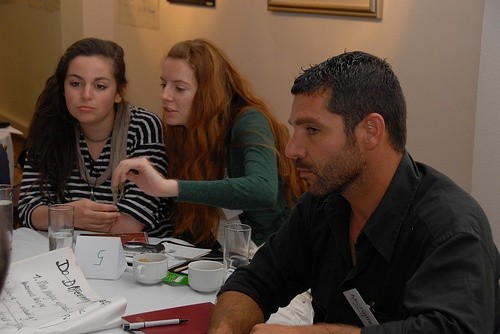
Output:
[0,184,13,294]
[132,253,168,284]
[188,260,227,292]
[48,203,74,252]
[224,223,251,277]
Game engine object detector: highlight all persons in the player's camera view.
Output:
[211,48,500,334]
[18,39,175,239]
[109,40,315,327]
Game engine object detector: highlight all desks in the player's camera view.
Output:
[0,226,314,334]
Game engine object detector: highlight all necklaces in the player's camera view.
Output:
[79,140,107,202]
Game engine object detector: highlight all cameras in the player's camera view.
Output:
[121,240,164,259]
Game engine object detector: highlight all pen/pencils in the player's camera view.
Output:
[122,318,191,330]
